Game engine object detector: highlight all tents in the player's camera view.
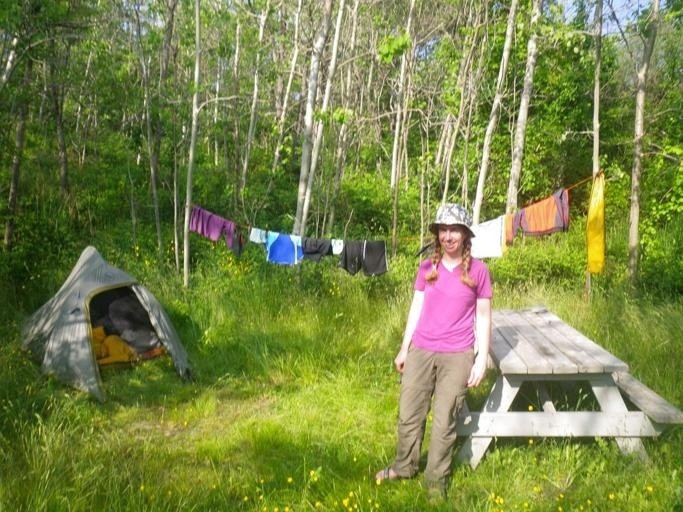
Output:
[19,245,194,406]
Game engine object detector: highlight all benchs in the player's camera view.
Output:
[452,397,494,472]
[610,368,682,471]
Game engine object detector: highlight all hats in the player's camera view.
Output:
[428,203,474,236]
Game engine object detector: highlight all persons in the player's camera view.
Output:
[374,203,493,501]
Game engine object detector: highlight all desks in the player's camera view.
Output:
[444,306,682,471]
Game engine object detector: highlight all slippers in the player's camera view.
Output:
[374,468,400,481]
